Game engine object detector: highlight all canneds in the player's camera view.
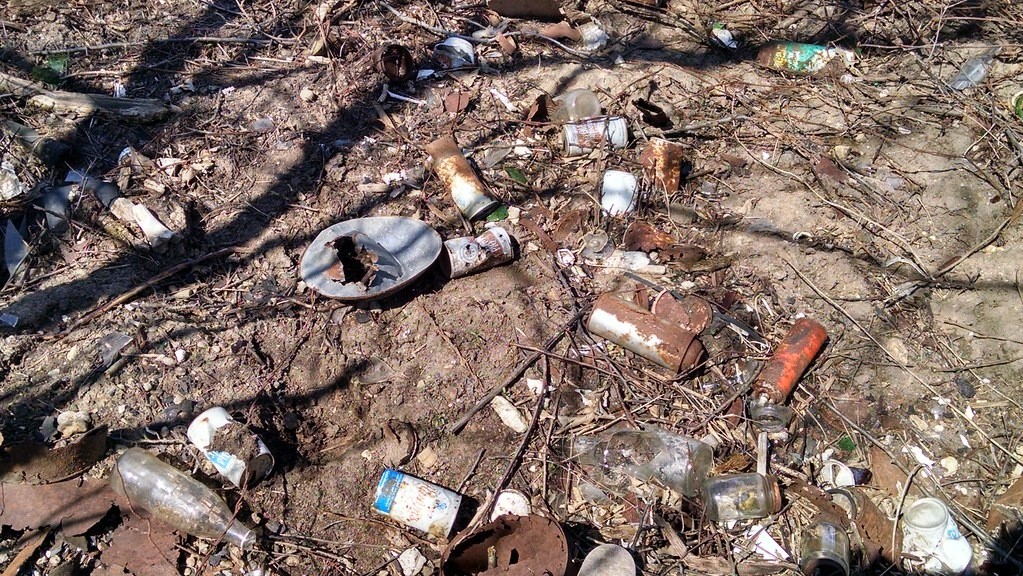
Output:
[186,406,275,490]
[371,469,461,540]
[559,117,628,156]
[904,498,973,575]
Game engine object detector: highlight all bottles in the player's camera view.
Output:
[585,291,705,374]
[556,115,627,155]
[752,317,829,406]
[109,446,257,550]
[574,431,850,576]
[755,38,854,77]
